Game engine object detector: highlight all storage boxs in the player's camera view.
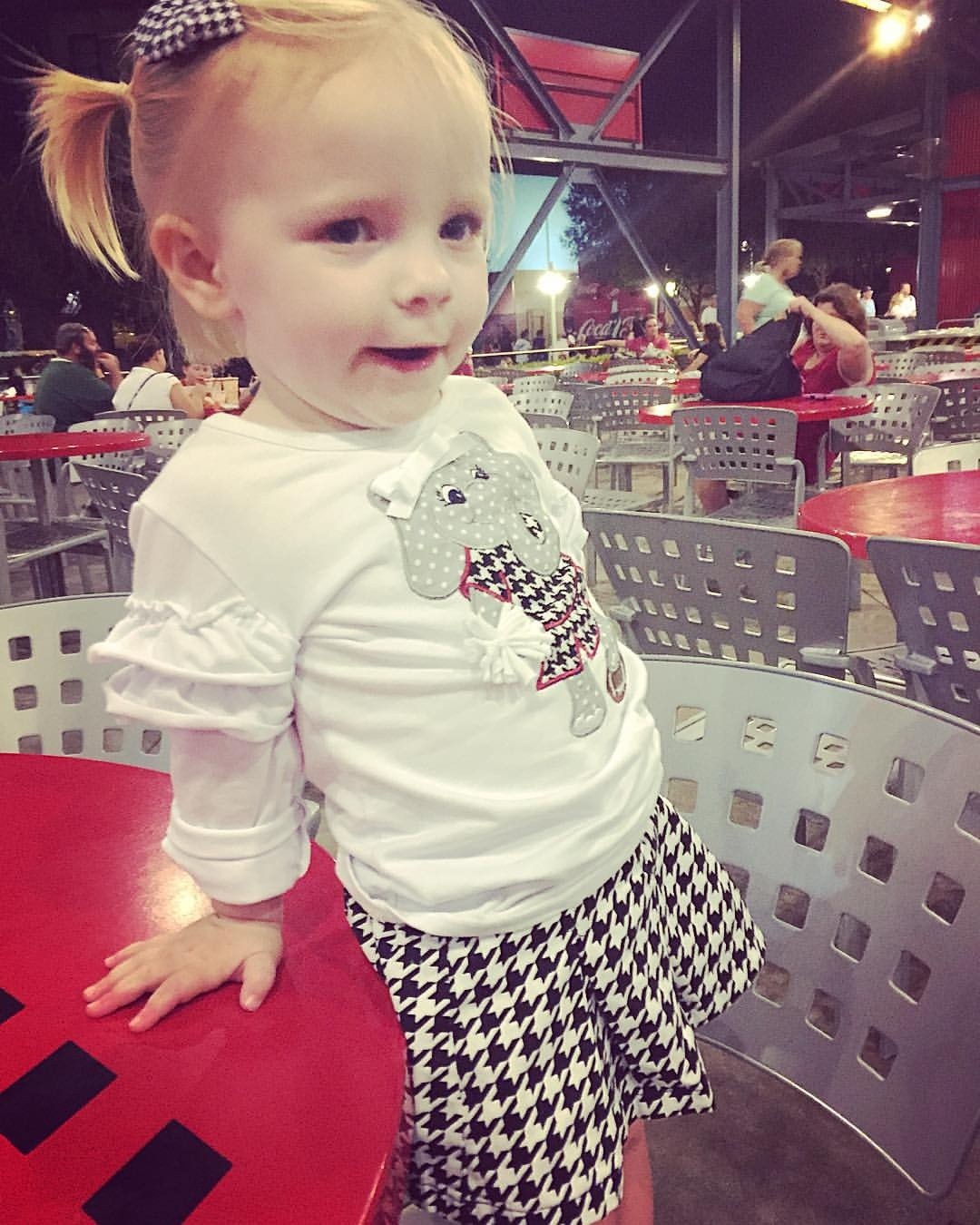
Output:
[487,26,644,146]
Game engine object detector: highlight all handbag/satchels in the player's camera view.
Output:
[701,308,804,399]
[639,341,670,359]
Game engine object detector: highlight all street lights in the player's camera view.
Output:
[536,272,568,363]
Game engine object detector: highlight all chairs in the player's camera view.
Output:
[623,648,980,1225]
[0,374,261,606]
[865,533,979,895]
[581,508,899,859]
[469,311,980,518]
[0,591,168,769]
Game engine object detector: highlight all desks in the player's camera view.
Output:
[0,747,411,1224]
[797,471,980,560]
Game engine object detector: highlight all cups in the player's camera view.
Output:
[204,377,241,410]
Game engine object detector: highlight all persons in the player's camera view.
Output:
[177,359,216,389]
[734,236,807,355]
[473,325,616,368]
[858,281,918,351]
[691,282,876,517]
[110,332,208,420]
[3,366,27,396]
[31,321,124,521]
[34,0,762,1225]
[698,291,720,325]
[624,313,671,366]
[680,323,728,375]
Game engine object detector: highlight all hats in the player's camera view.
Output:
[860,286,872,293]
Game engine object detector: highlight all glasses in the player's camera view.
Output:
[866,291,873,293]
